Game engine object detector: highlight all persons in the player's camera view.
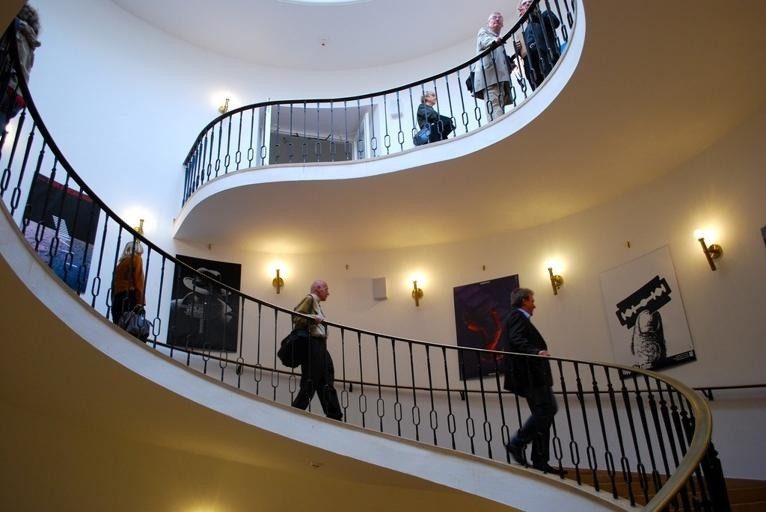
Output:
[0,5,41,142]
[277,279,343,422]
[413,90,456,146]
[466,12,517,123]
[512,0,561,92]
[633,308,666,366]
[499,288,568,474]
[168,266,236,351]
[111,241,149,344]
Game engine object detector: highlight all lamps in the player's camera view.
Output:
[694,228,722,274]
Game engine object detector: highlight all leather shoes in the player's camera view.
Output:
[533,462,569,475]
[505,445,527,466]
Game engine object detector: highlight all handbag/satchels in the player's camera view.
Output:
[277,327,309,368]
[119,304,150,343]
[414,127,429,144]
